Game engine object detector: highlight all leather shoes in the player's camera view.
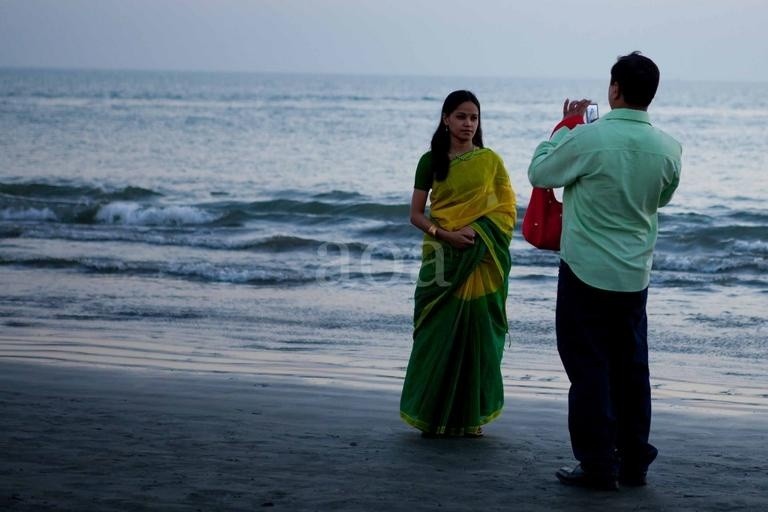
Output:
[554,461,649,494]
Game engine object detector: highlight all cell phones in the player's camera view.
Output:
[585,104,598,122]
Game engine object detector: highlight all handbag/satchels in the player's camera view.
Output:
[521,186,564,251]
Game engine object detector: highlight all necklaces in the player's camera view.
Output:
[455,144,475,161]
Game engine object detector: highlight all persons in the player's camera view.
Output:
[400,90,518,438]
[529,52,682,493]
[590,108,596,120]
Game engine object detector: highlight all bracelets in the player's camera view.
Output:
[428,224,437,236]
